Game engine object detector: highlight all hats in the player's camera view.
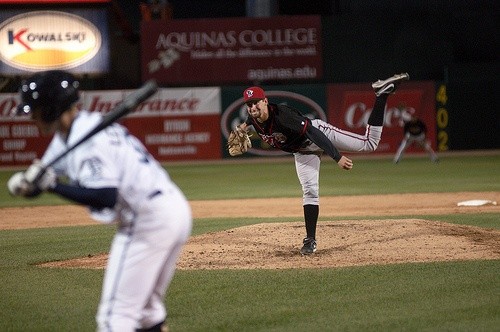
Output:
[244,86,265,102]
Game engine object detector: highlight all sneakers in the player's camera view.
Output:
[372,73,409,97]
[300,238,318,255]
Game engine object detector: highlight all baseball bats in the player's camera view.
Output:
[8,81,159,198]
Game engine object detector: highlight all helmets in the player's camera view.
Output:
[12,70,79,117]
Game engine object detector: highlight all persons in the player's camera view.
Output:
[7,70,193,332]
[228,73,409,253]
[394,106,440,163]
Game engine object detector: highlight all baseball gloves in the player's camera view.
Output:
[226,125,254,157]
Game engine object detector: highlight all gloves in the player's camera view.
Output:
[7,159,60,200]
